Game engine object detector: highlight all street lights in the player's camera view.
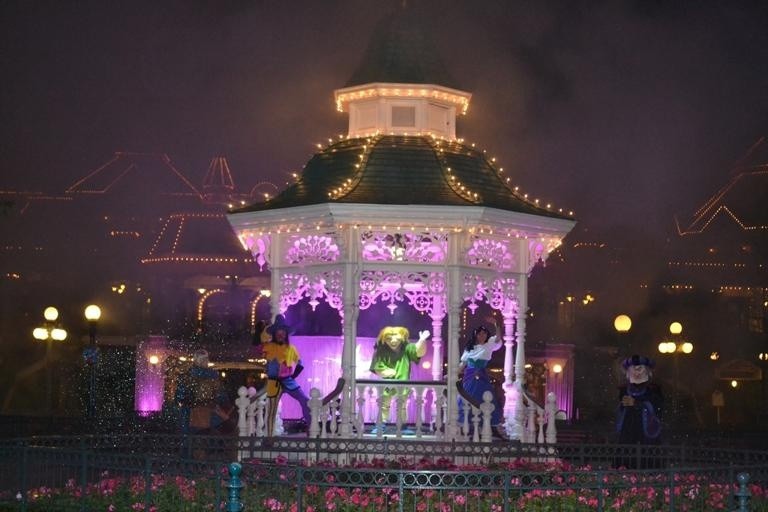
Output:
[614,314,632,396]
[659,321,694,414]
[85,305,101,416]
[552,362,563,400]
[33,307,67,416]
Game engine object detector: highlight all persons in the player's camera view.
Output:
[614,353,664,466]
[370,327,429,435]
[253,313,320,435]
[443,322,503,436]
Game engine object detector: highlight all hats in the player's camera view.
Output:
[265,314,297,336]
